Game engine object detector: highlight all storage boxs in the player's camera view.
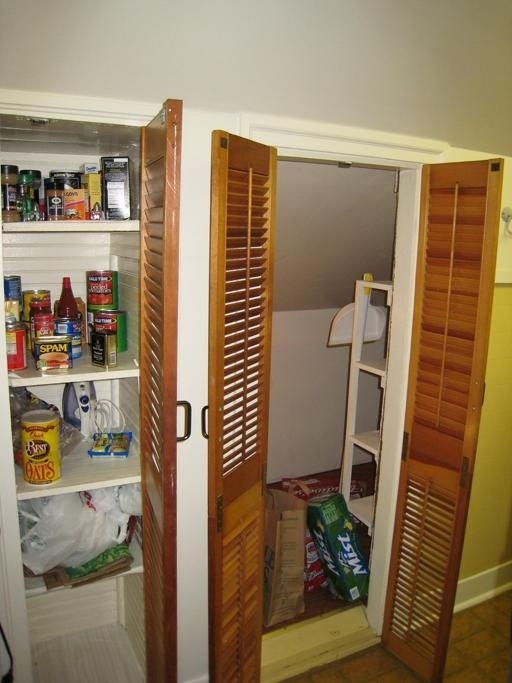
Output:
[306,492,369,604]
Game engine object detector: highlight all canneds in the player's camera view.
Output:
[19,409,62,486]
[4,269,128,371]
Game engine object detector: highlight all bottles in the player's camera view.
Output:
[58,277,77,321]
[2,165,62,222]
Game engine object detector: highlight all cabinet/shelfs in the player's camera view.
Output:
[338,279,394,536]
[0,88,163,644]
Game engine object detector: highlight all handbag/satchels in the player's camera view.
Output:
[263,477,310,627]
